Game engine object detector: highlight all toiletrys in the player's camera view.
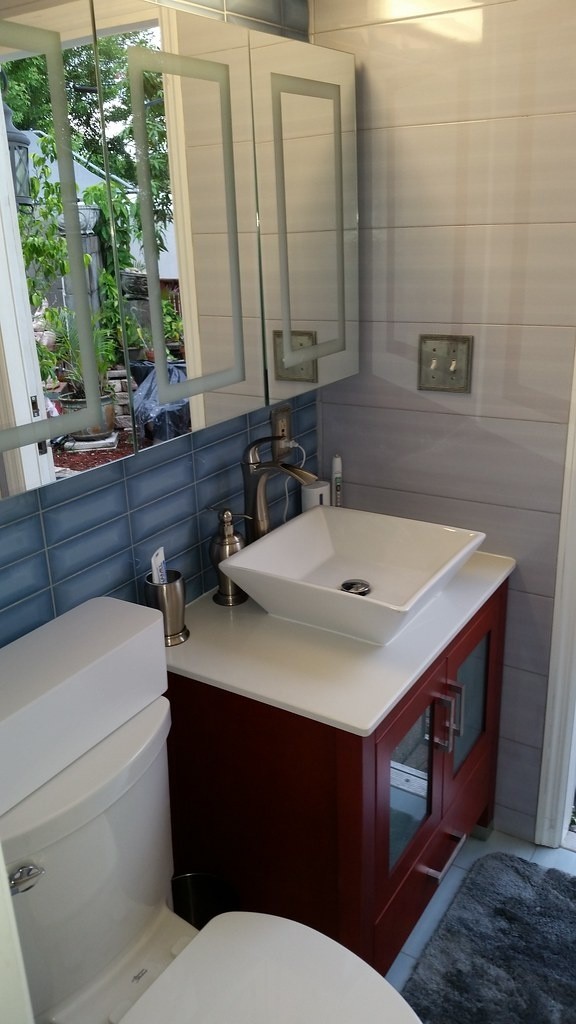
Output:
[151,547,167,585]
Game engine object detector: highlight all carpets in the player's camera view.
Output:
[398,852,576,1024]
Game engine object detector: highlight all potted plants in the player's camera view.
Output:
[125,314,145,359]
[43,295,124,441]
[161,298,184,351]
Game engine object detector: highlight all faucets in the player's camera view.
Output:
[239,435,318,544]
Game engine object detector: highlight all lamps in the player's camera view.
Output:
[0,68,34,214]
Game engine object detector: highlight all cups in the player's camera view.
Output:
[145,570,189,647]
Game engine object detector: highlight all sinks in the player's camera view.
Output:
[220,505,486,644]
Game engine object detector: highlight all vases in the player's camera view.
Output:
[119,272,148,300]
[49,205,99,235]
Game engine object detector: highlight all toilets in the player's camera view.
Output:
[0,595,423,1024]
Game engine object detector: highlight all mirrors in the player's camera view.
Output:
[0,0,359,486]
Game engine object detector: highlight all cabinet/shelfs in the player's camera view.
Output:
[167,575,505,975]
